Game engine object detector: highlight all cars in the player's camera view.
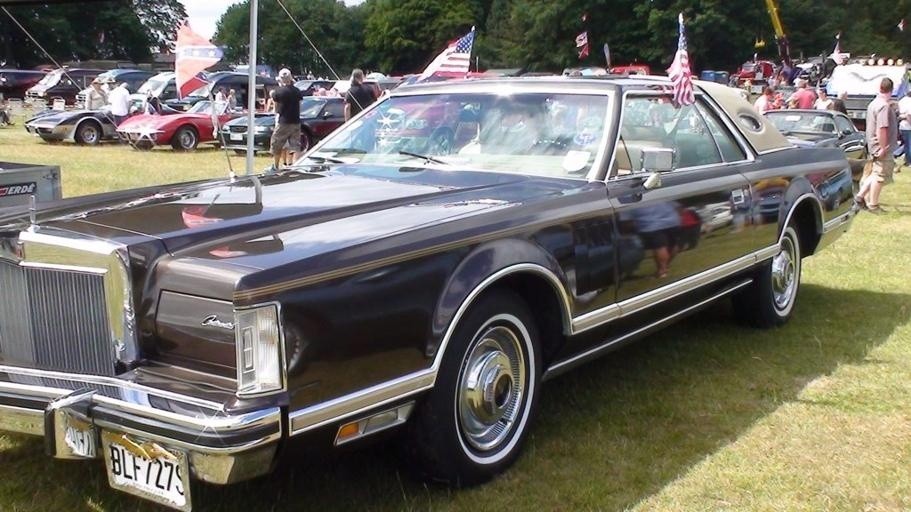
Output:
[0,66,473,155]
[1,75,861,512]
[481,67,648,76]
[701,54,838,90]
[755,109,867,159]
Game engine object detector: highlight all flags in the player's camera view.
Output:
[414,31,476,82]
[174,20,226,104]
[666,15,700,108]
[573,30,591,60]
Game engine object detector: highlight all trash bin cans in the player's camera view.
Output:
[701,69,729,84]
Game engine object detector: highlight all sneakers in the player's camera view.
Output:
[265,166,275,172]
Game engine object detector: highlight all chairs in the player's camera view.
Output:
[618,126,670,176]
[459,104,546,155]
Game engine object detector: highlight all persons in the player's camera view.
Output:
[215,88,227,103]
[269,67,305,172]
[730,56,911,169]
[87,78,134,146]
[307,68,342,99]
[145,94,184,116]
[273,77,298,166]
[265,89,276,112]
[228,90,239,110]
[343,67,379,153]
[614,196,716,283]
[853,77,900,216]
[1,76,14,129]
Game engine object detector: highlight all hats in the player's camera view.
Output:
[279,68,292,79]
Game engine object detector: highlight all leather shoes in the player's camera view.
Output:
[869,205,886,216]
[853,197,869,212]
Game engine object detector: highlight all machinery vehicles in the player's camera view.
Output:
[754,0,793,68]
